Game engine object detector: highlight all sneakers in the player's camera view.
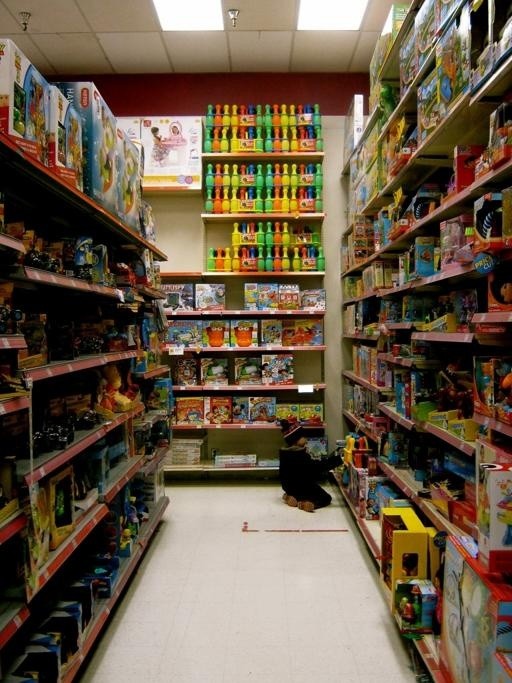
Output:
[283,493,298,507]
[297,500,315,512]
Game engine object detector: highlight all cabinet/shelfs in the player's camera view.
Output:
[201,122,325,225]
[0,131,171,683]
[158,271,326,472]
[329,0,512,682]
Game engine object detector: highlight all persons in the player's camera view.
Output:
[277,418,342,514]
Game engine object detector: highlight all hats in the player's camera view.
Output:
[280,418,305,445]
